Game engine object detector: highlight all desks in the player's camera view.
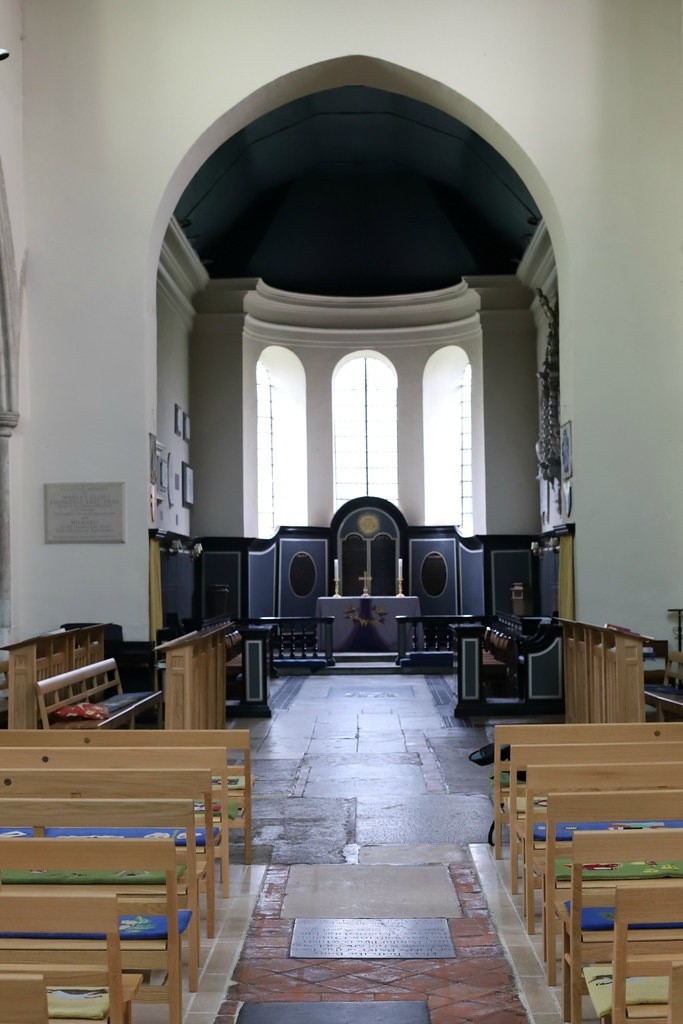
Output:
[317,596,424,651]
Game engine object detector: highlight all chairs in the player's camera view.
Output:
[479,625,511,682]
[187,611,243,699]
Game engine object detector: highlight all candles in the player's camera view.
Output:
[332,559,341,598]
[396,558,405,599]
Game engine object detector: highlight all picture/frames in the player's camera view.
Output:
[148,404,196,514]
[559,419,573,481]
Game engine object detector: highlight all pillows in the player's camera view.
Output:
[56,702,109,719]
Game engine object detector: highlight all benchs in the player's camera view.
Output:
[35,657,162,730]
[0,726,266,1024]
[645,651,683,723]
[490,718,683,1024]
